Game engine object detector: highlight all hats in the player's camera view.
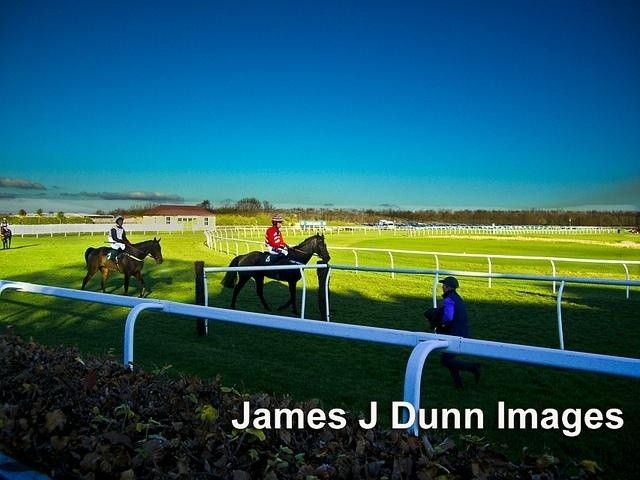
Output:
[271,214,283,222]
[437,276,459,289]
[115,215,124,223]
[3,217,7,220]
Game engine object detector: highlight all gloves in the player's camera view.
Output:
[122,240,132,246]
[277,246,288,256]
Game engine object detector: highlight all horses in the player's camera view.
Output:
[81,237,163,297]
[220,233,331,312]
[0,226,12,250]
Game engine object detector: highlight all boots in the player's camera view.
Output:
[451,362,480,389]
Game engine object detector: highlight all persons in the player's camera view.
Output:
[264,214,290,265]
[0,217,11,236]
[107,214,133,265]
[423,276,483,390]
